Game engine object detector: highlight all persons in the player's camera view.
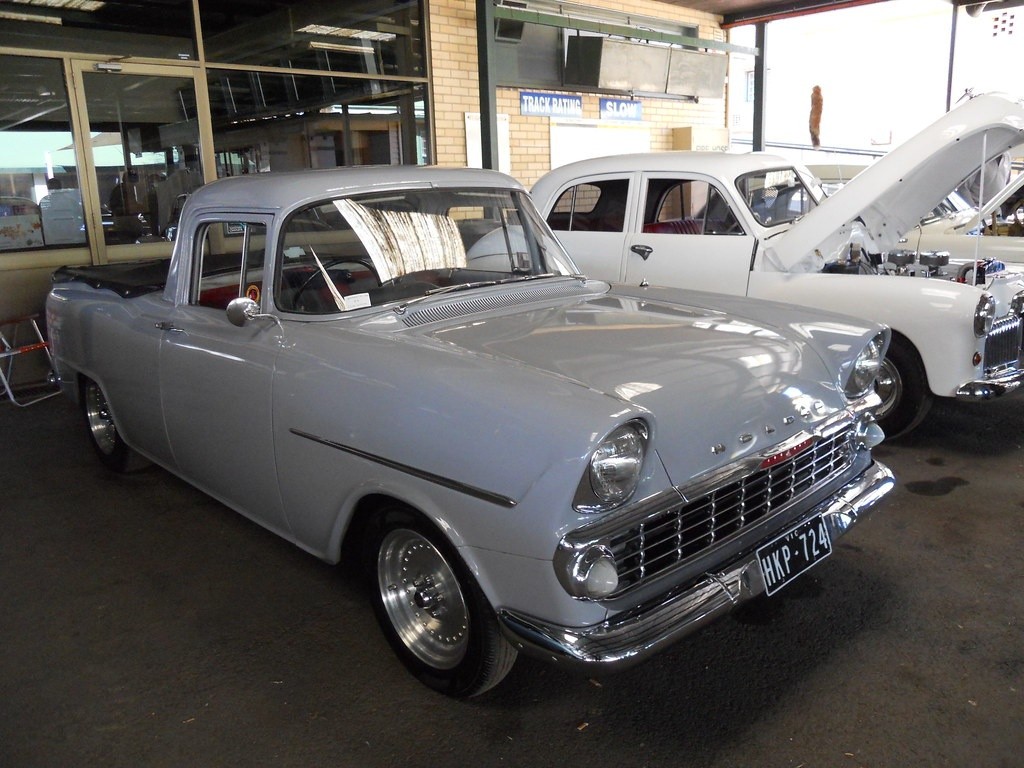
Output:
[810,86,823,146]
[958,151,1011,208]
[37,178,63,245]
[110,171,166,243]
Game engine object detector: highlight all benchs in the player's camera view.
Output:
[644,219,729,234]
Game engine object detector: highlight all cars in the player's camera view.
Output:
[463,84,1023,444]
[42,161,898,701]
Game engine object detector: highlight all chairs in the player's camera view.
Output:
[0,313,64,408]
